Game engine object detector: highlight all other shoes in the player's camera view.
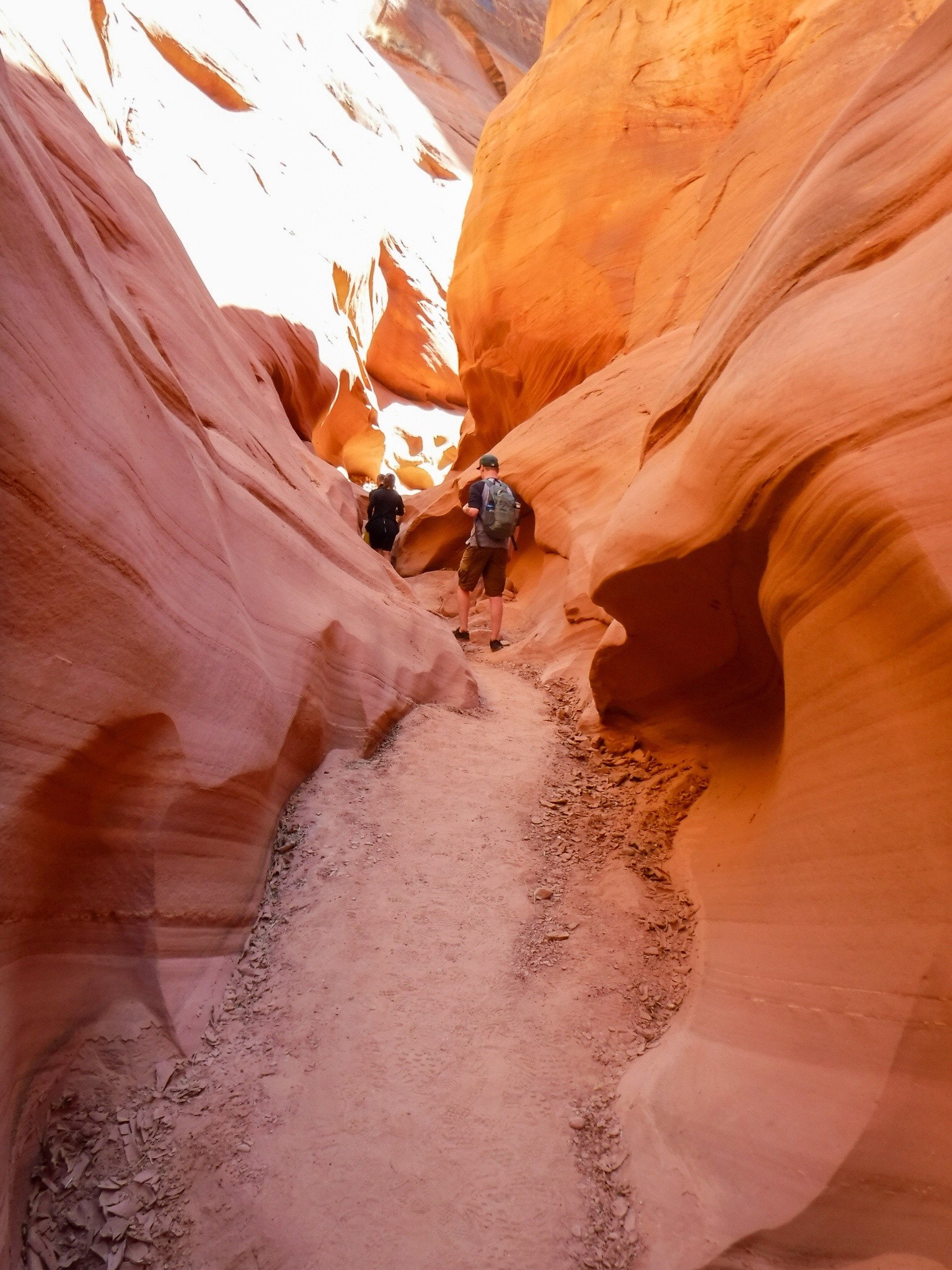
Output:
[452,626,470,642]
[490,638,504,652]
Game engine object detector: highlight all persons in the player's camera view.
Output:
[365,473,405,563]
[452,454,515,652]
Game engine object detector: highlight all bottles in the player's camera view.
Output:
[513,502,521,524]
[485,500,495,526]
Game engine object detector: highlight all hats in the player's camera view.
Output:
[384,473,396,481]
[476,455,499,470]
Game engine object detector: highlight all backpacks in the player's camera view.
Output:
[474,479,522,551]
